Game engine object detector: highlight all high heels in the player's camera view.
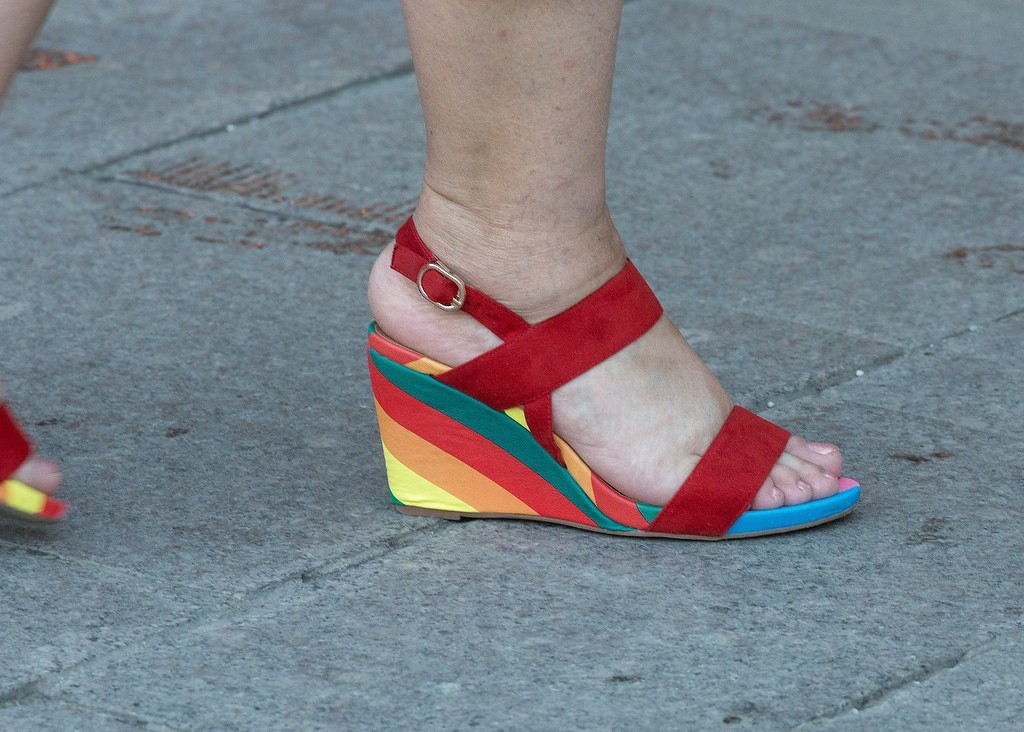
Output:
[367,215,861,540]
[0,402,68,520]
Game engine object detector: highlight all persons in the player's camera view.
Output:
[0,0,861,540]
